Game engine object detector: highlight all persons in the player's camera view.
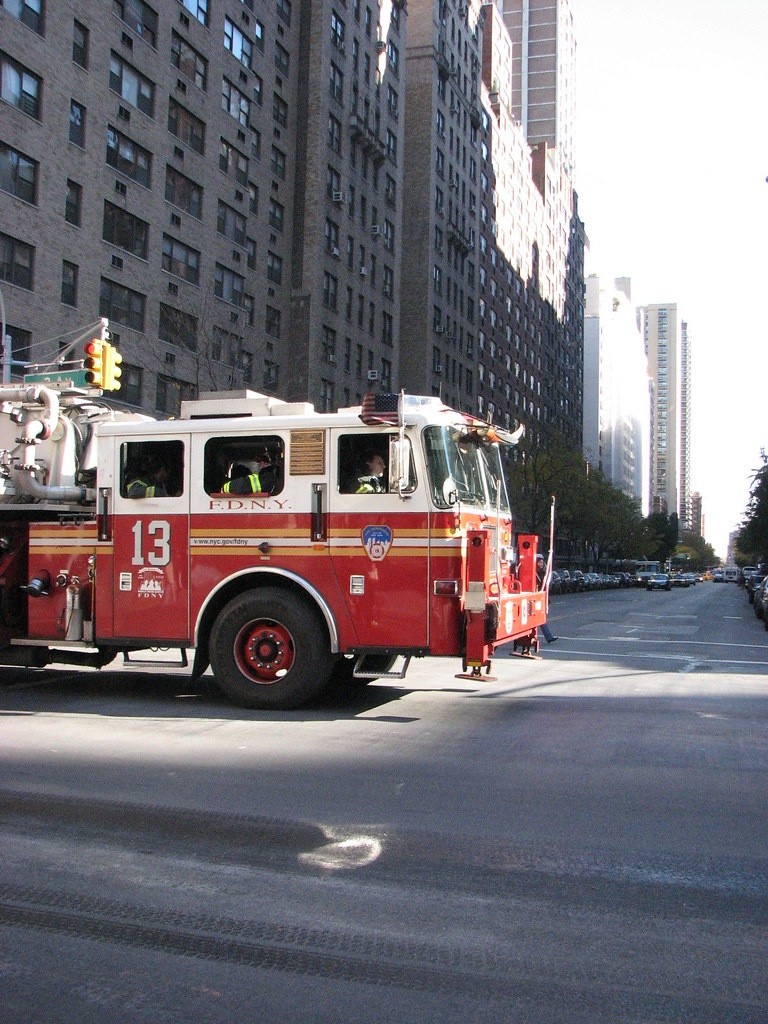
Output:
[205,437,285,496]
[120,441,184,498]
[346,452,387,494]
[535,554,558,644]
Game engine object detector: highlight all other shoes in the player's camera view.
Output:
[548,638,558,643]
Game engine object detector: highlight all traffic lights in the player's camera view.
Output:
[83,338,102,387]
[104,346,122,391]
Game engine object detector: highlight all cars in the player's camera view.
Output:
[549,566,768,631]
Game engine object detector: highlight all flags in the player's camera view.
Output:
[362,391,403,425]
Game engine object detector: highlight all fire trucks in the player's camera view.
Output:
[0,287,558,709]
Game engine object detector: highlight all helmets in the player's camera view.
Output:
[140,453,165,473]
[263,436,284,451]
